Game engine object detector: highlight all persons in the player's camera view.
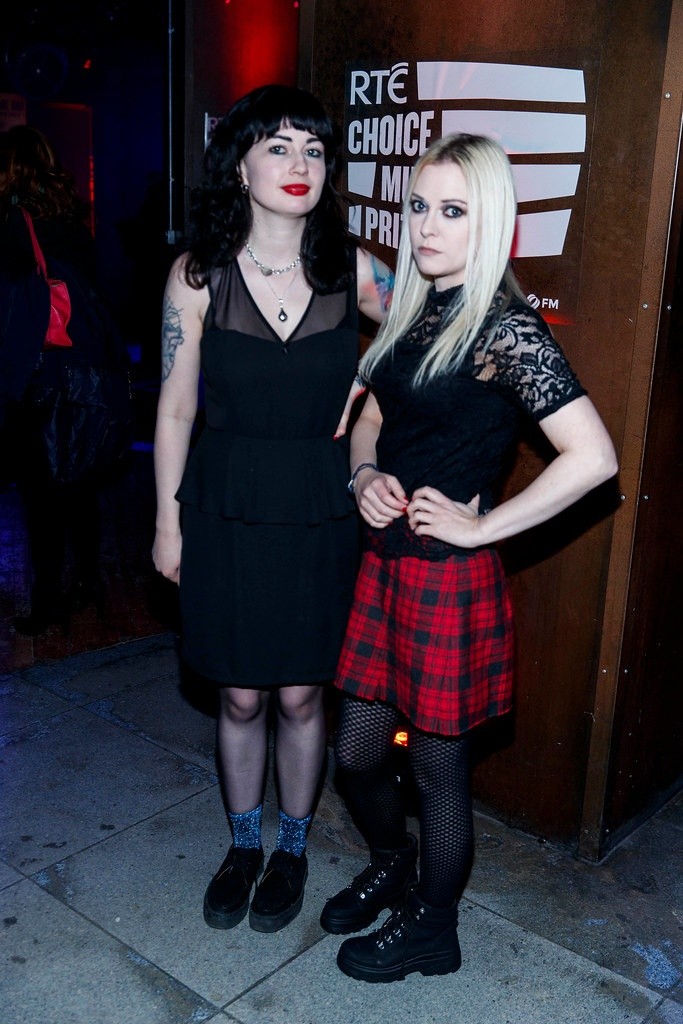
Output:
[321,130,619,986]
[151,80,395,936]
[0,125,134,637]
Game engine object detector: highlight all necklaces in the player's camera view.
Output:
[242,239,314,324]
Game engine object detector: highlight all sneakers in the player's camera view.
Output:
[249,851,310,933]
[337,884,462,984]
[319,833,418,935]
[203,842,265,930]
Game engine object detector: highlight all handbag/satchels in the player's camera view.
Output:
[42,277,73,350]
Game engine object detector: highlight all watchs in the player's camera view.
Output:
[347,461,380,496]
